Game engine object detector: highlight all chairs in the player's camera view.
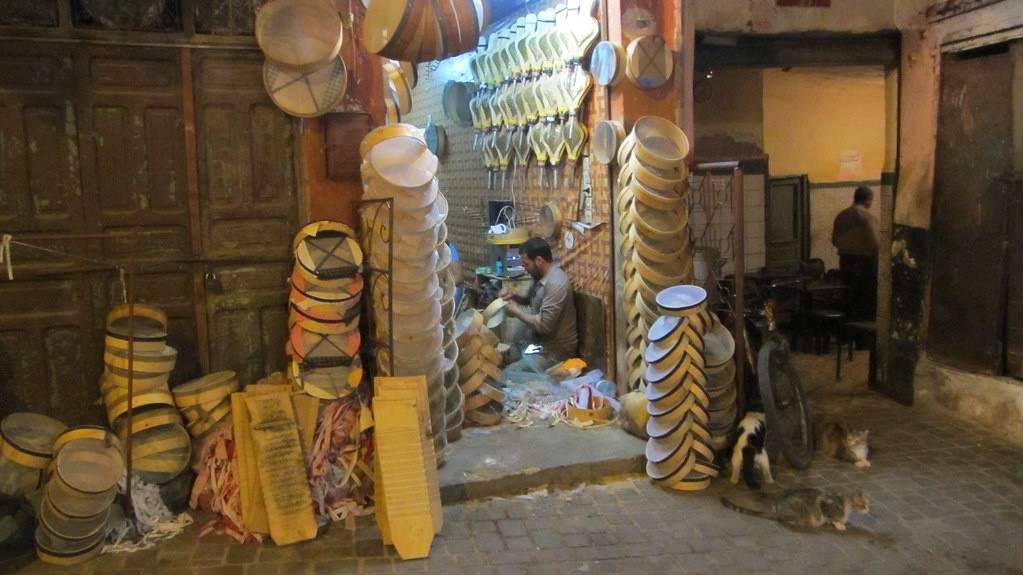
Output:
[766,259,856,355]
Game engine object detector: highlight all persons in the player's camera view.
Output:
[831,185,879,352]
[502,237,579,377]
[445,239,464,313]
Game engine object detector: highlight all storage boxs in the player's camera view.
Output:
[688,175,765,276]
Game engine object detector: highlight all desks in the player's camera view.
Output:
[833,320,877,388]
